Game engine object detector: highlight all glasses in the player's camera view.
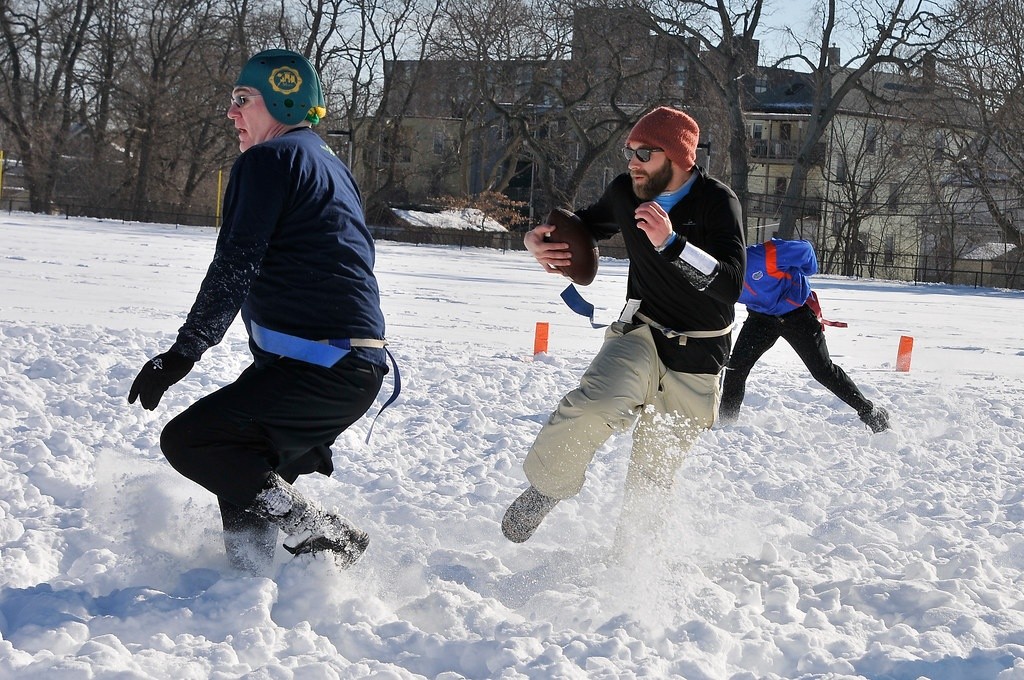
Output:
[622,146,664,163]
[230,94,264,108]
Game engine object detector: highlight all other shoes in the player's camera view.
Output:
[866,408,888,435]
[501,486,560,543]
[283,513,371,573]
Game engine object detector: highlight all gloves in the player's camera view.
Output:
[127,341,199,411]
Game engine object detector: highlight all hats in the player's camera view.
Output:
[627,106,700,172]
[238,49,327,125]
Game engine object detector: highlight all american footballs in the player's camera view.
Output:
[542,206,600,287]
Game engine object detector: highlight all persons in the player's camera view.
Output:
[719,237,892,433]
[128,49,401,572]
[501,106,748,549]
